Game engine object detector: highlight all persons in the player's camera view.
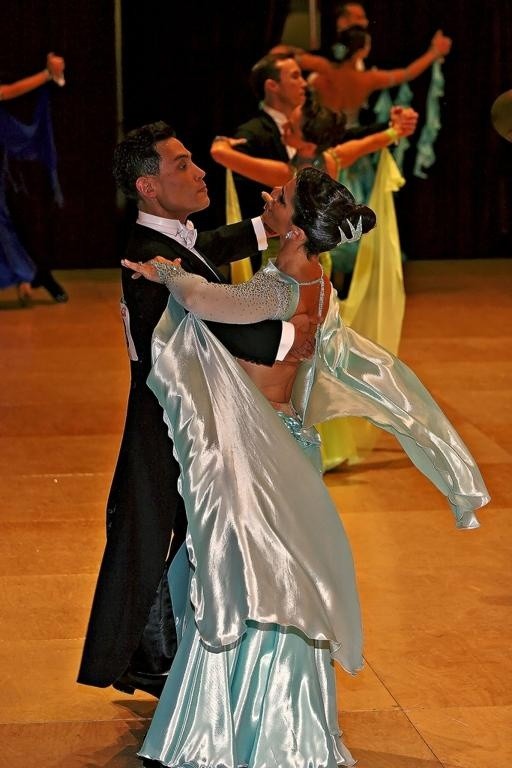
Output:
[0,51,69,306]
